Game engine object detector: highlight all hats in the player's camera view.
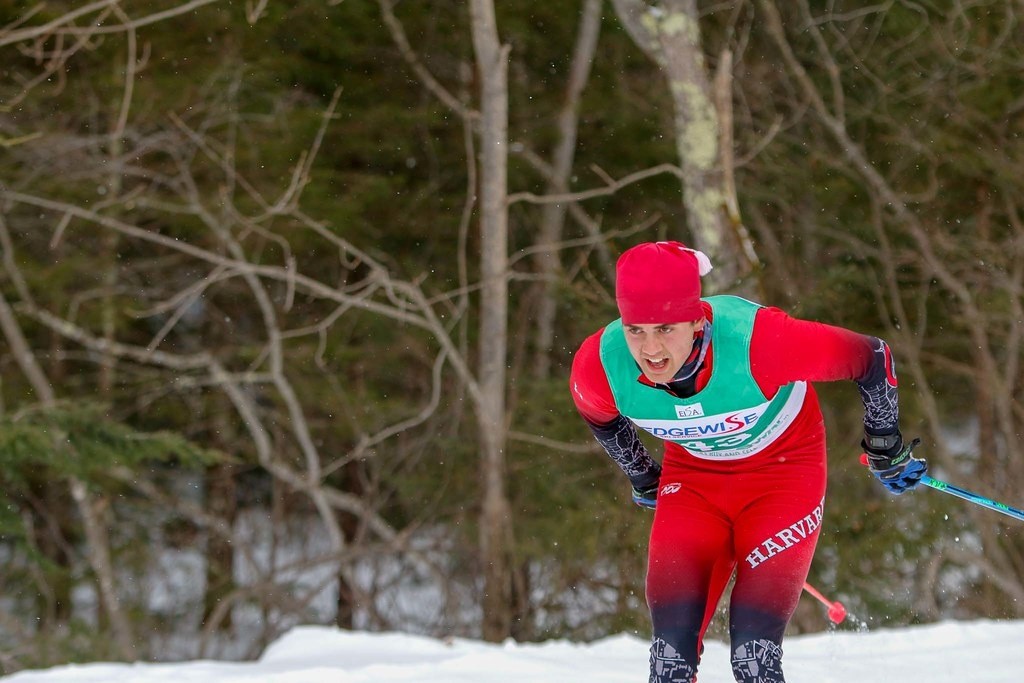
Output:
[615,240,706,324]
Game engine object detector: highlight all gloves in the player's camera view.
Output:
[859,430,928,496]
[629,463,662,510]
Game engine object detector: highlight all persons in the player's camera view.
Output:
[570,239,929,683]
